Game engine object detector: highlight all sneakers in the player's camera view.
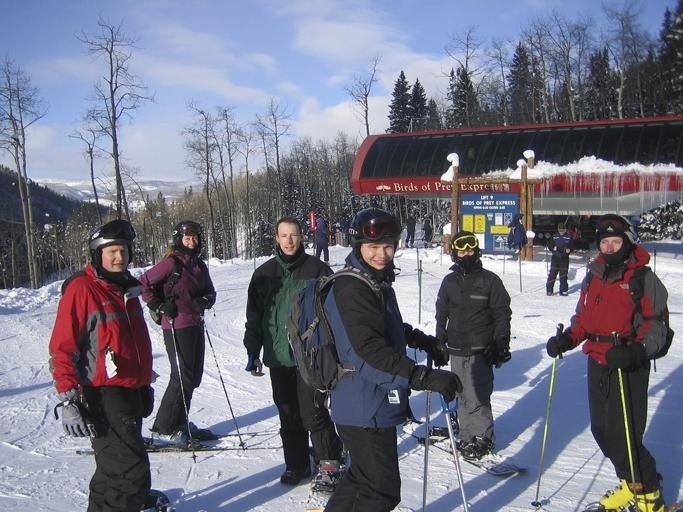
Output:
[182,422,199,434]
[429,411,459,436]
[462,436,494,459]
[152,431,188,447]
[281,455,311,485]
[560,292,567,296]
[547,291,553,296]
[313,459,341,492]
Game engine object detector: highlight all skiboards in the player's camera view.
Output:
[73,429,282,456]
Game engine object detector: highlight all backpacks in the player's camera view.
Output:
[629,265,674,360]
[149,254,182,325]
[286,267,385,389]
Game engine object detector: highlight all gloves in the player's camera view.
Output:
[605,342,647,371]
[56,388,90,437]
[252,359,265,376]
[547,330,576,358]
[409,363,463,404]
[408,328,449,367]
[143,385,153,418]
[147,299,177,318]
[485,338,510,368]
[193,295,211,313]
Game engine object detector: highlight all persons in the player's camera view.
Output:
[434,230,513,460]
[544,221,573,296]
[545,213,668,512]
[242,216,347,495]
[137,220,216,451]
[312,212,448,267]
[324,207,463,512]
[46,219,156,512]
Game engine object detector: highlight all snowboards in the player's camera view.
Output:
[304,447,351,509]
[402,418,527,476]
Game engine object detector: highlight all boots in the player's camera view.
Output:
[599,478,665,512]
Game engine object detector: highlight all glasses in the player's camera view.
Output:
[89,220,136,243]
[172,223,201,236]
[450,234,479,251]
[347,217,403,240]
[598,214,630,232]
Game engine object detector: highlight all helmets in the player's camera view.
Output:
[89,226,133,255]
[596,213,634,246]
[451,232,482,269]
[173,221,201,247]
[349,208,402,252]
